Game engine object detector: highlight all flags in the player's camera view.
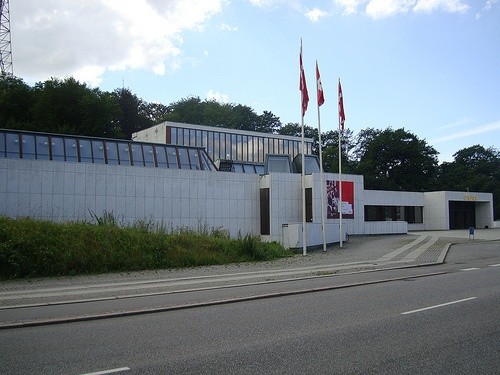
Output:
[339,83,346,131]
[316,64,325,106]
[299,48,308,116]
